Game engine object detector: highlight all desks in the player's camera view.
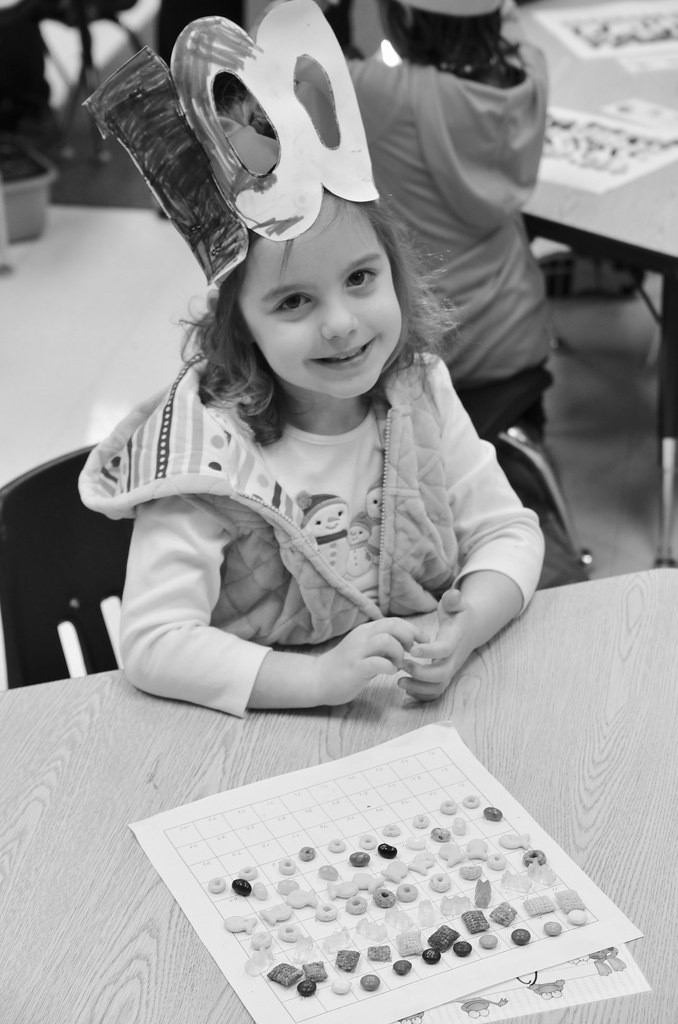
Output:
[0,567,678,1024]
[0,202,210,500]
[518,0,678,572]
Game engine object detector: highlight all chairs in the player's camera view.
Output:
[463,364,597,575]
[0,424,143,688]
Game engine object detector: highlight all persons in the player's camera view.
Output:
[1,1,646,537]
[75,98,546,717]
